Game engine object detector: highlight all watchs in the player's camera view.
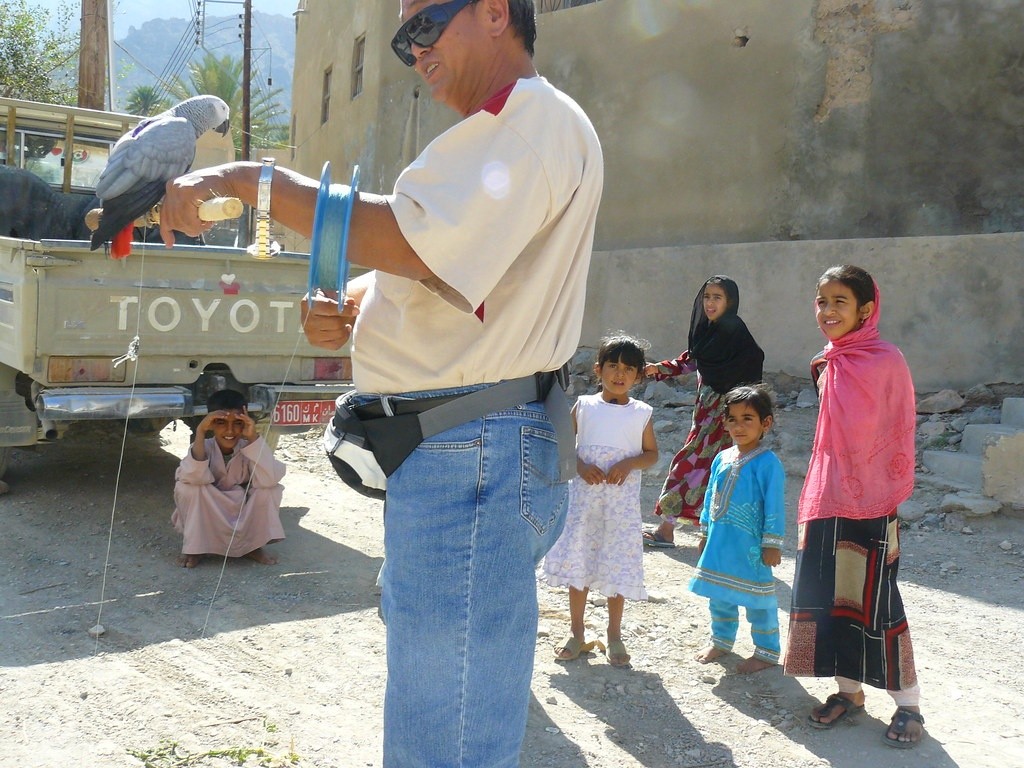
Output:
[247,158,280,260]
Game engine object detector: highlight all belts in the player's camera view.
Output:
[347,393,466,422]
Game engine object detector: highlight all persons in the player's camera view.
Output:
[543,339,659,668]
[169,389,287,568]
[639,273,765,551]
[159,0,606,768]
[688,384,784,676]
[794,263,925,750]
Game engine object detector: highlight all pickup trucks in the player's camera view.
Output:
[0,97,355,486]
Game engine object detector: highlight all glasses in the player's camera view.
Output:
[391,1,472,67]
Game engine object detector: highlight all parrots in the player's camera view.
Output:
[88,95,231,259]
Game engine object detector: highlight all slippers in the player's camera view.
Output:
[596,637,630,667]
[882,708,925,748]
[806,694,865,729]
[552,635,596,661]
[642,530,676,547]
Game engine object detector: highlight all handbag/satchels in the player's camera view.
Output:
[322,360,580,500]
